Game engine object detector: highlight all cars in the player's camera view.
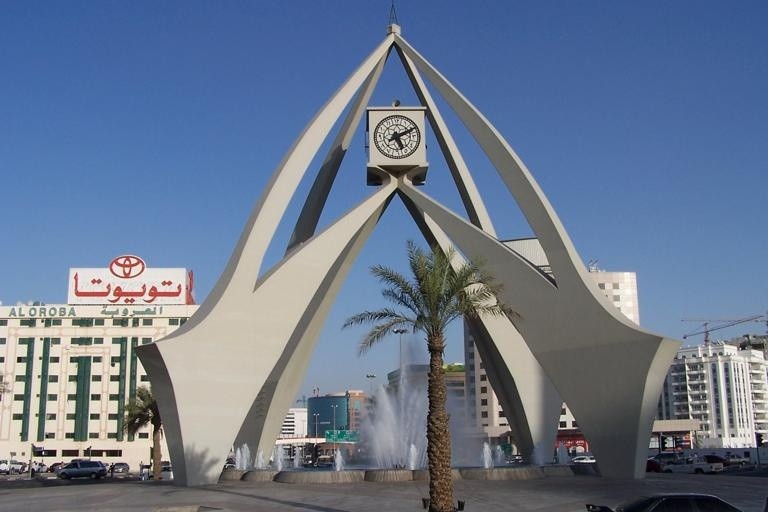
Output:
[584,492,746,512]
[645,450,750,476]
[0,458,174,483]
[570,456,596,463]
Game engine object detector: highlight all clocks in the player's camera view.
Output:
[372,114,421,159]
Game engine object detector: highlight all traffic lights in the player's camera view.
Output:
[674,437,680,449]
[756,433,764,448]
[661,435,668,451]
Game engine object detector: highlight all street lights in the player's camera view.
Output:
[86,445,92,461]
[366,372,377,411]
[29,442,35,479]
[330,403,336,467]
[392,327,409,373]
[313,412,320,445]
